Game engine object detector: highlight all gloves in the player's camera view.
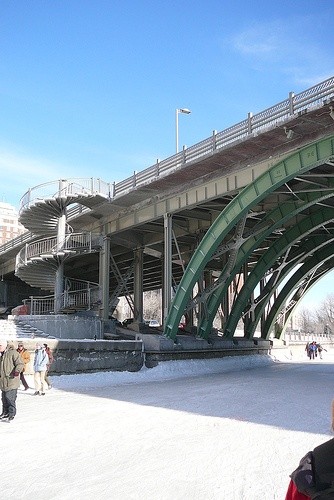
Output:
[13,372,19,376]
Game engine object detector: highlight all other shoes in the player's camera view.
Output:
[2,416,15,422]
[41,393,45,396]
[24,386,30,391]
[48,384,53,389]
[34,391,40,396]
[0,413,9,419]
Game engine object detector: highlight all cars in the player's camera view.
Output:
[0,305,15,319]
[122,318,160,327]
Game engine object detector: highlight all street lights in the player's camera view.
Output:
[175,107,192,153]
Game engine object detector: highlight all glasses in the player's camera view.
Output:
[18,345,23,347]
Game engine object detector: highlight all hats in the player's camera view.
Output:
[18,342,23,347]
[0,339,7,346]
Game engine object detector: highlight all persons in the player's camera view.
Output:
[284,397,334,500]
[32,343,49,396]
[306,341,326,360]
[0,337,24,420]
[40,344,54,389]
[14,342,30,390]
